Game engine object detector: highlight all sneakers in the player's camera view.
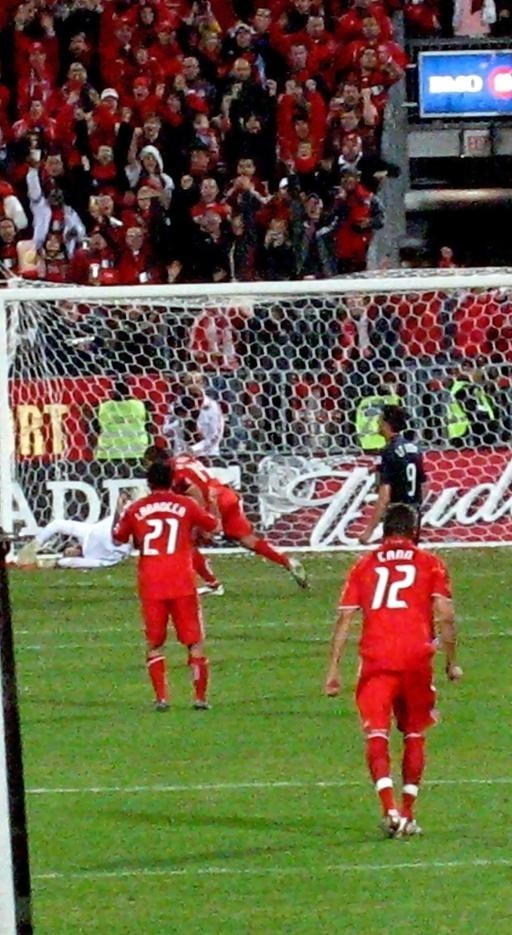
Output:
[155,698,169,711]
[383,809,420,839]
[288,559,308,585]
[194,700,210,709]
[196,583,222,596]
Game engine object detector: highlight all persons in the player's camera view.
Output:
[143,444,309,596]
[446,356,512,448]
[323,502,464,838]
[87,371,223,466]
[356,376,401,456]
[112,462,222,709]
[359,402,429,547]
[1,1,512,372]
